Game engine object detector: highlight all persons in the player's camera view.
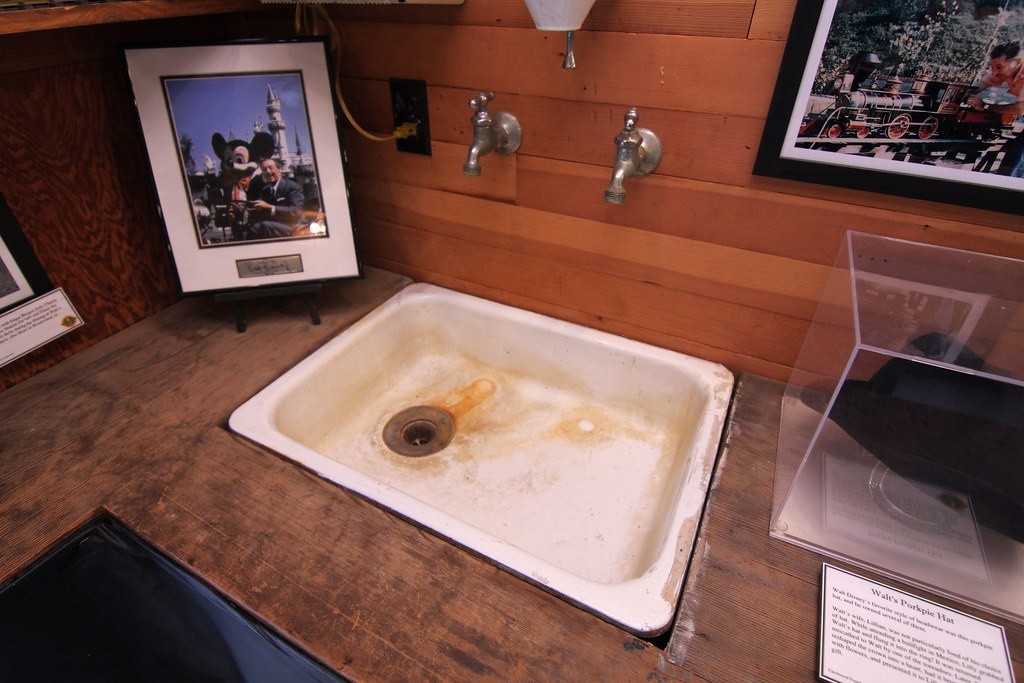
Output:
[222,160,305,239]
[966,39,1024,178]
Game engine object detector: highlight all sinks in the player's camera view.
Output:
[227,290,739,645]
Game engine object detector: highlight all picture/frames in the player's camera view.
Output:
[117,35,366,331]
[752,0,1024,215]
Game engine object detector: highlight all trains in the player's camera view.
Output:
[796,51,1024,167]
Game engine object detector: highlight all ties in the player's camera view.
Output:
[272,189,275,197]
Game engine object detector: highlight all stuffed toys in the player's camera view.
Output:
[202,131,275,209]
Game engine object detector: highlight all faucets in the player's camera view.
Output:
[603,106,663,206]
[461,90,524,177]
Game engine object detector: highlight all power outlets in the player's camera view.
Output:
[388,78,431,159]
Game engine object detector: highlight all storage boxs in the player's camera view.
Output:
[766,231,1024,621]
